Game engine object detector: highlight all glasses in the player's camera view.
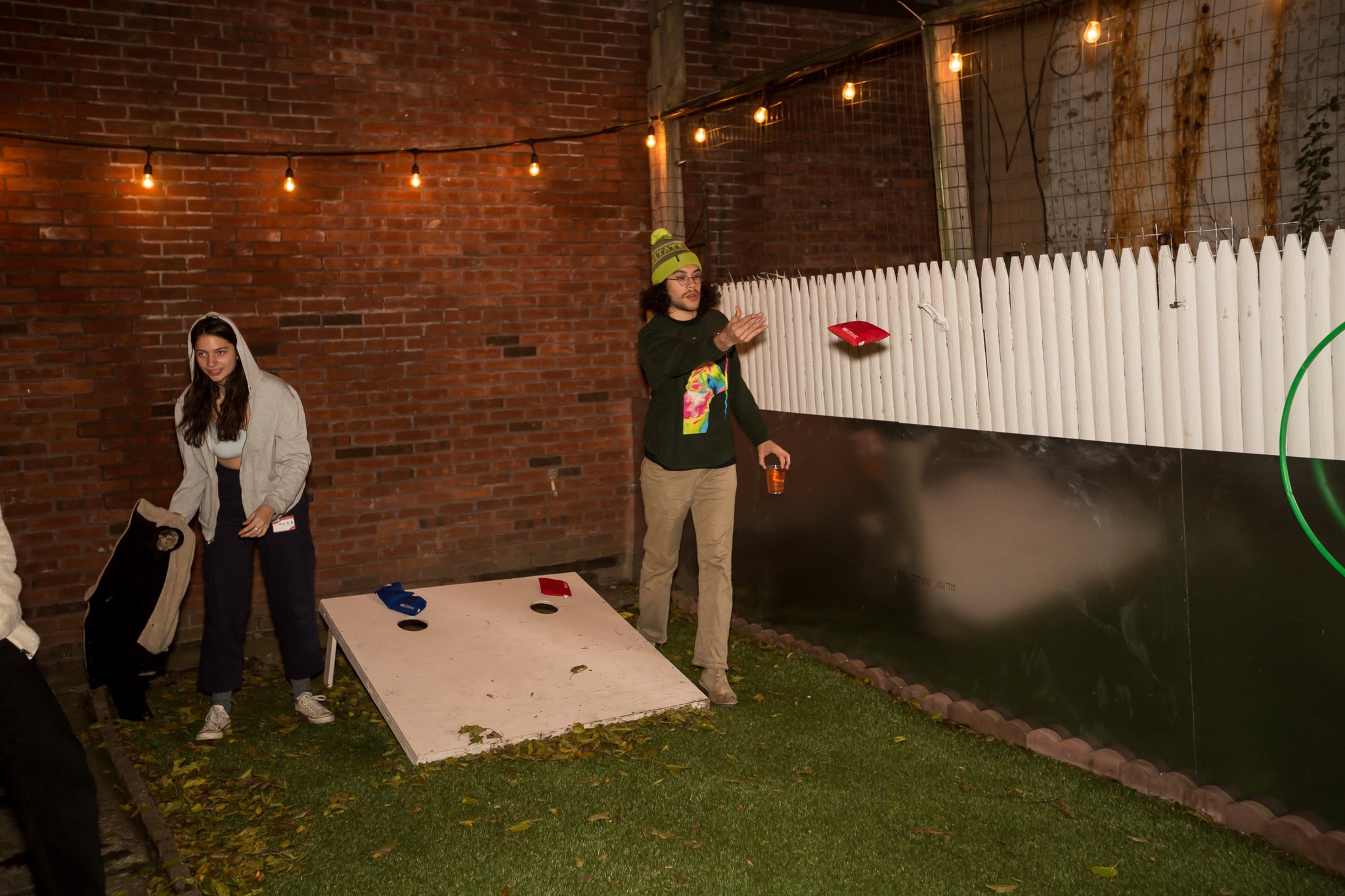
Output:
[665,273,704,285]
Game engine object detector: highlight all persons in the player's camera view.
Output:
[163,310,336,740]
[0,507,110,896]
[628,228,792,704]
[845,418,940,633]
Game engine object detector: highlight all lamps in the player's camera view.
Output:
[754,89,775,122]
[527,141,540,176]
[694,109,707,143]
[284,156,297,193]
[141,150,155,189]
[645,121,658,148]
[1083,0,1103,44]
[948,21,964,72]
[408,152,422,188]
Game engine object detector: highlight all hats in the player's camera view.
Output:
[651,227,701,286]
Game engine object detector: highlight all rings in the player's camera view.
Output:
[254,527,258,531]
[258,529,262,533]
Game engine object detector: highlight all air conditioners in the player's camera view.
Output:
[841,58,858,100]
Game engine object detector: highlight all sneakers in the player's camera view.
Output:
[294,692,336,725]
[698,666,738,704]
[196,705,232,741]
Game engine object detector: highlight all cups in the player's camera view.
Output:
[766,465,786,495]
